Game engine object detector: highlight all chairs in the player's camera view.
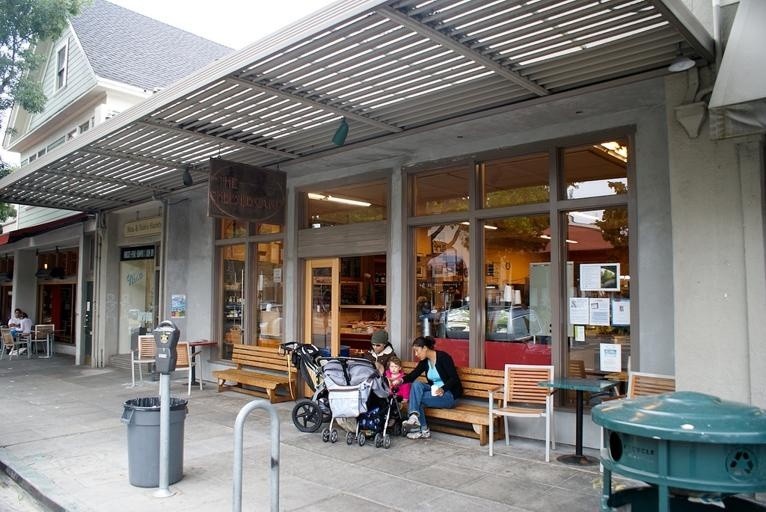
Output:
[0,324,55,360]
[487,364,556,463]
[600,371,675,472]
[131,335,157,388]
[175,341,203,395]
[567,360,605,405]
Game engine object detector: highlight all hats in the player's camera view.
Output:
[369,329,390,345]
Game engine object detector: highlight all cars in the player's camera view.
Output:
[226,302,244,324]
[257,305,283,328]
[444,303,544,340]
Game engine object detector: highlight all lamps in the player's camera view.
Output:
[668,41,696,72]
[182,167,194,187]
[308,193,373,208]
[332,117,350,146]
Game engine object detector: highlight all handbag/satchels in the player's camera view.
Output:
[323,375,374,419]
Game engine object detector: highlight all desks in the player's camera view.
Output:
[537,376,622,466]
[181,341,218,386]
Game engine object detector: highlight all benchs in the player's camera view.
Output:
[212,344,298,404]
[400,361,505,446]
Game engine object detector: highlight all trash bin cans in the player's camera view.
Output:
[591,391,766,512]
[120,397,189,487]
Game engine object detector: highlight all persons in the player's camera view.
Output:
[7,308,22,335]
[363,329,402,376]
[392,335,464,439]
[14,311,31,335]
[384,355,412,404]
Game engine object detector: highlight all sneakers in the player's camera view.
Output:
[401,415,422,429]
[406,426,431,439]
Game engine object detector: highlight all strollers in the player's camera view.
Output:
[280,341,408,449]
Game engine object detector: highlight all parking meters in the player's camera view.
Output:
[153,320,180,499]
